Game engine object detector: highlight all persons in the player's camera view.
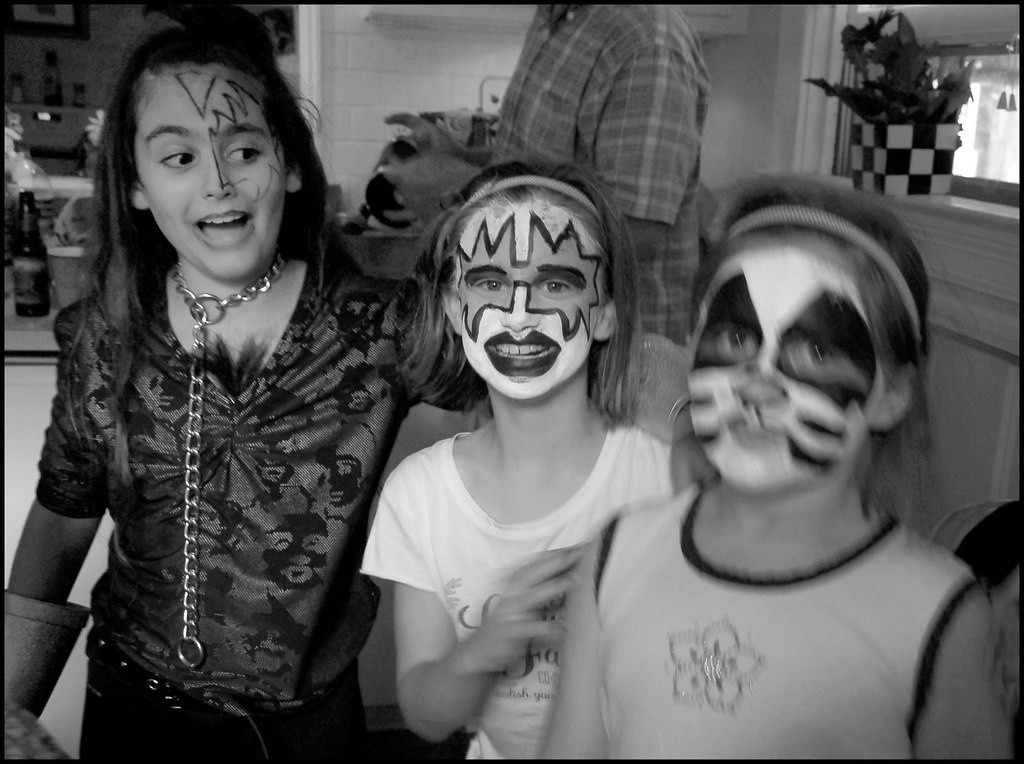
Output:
[359,160,692,759]
[375,3,711,347]
[5,27,723,760]
[539,175,1013,759]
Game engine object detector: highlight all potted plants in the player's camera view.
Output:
[806,6,974,196]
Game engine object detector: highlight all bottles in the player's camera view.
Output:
[42,53,63,105]
[12,192,50,317]
[12,143,56,237]
[11,74,25,102]
[73,72,85,106]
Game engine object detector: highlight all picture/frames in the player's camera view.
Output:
[4,5,91,41]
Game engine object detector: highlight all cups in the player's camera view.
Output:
[46,247,84,308]
[365,136,418,229]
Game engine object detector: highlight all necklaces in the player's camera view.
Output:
[171,248,284,669]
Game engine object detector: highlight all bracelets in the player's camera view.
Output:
[666,393,690,425]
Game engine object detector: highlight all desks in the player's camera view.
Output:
[5,281,62,351]
